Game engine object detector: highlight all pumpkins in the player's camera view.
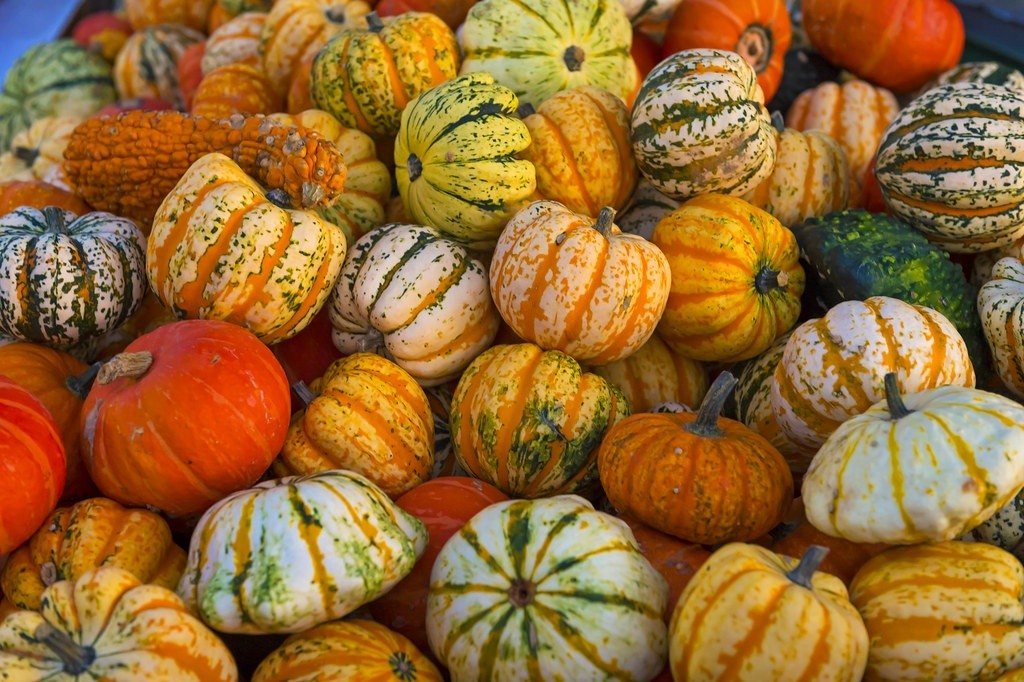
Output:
[0,0,1024,682]
